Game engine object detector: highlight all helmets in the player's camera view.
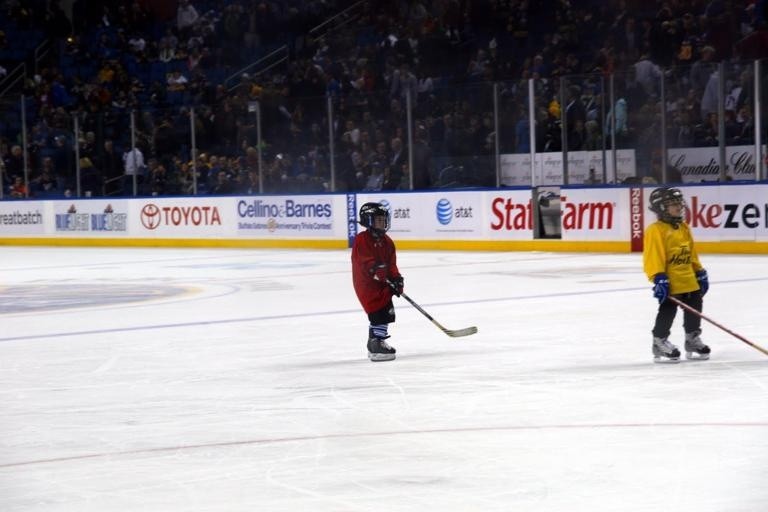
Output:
[649,188,683,222]
[359,203,391,238]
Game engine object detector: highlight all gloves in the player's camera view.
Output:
[696,268,709,297]
[652,275,668,304]
[391,274,404,298]
[369,263,390,287]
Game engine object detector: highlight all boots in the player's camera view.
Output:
[653,336,678,355]
[367,327,395,354]
[685,333,711,353]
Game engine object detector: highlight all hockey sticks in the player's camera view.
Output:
[373,274,477,337]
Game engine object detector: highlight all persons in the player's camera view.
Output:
[643,187,711,357]
[351,203,404,355]
